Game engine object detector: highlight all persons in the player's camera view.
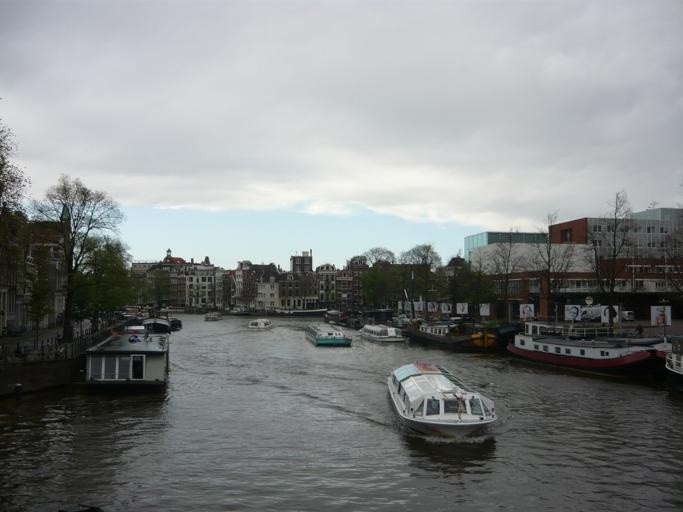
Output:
[431,303,435,310]
[448,304,450,311]
[569,306,579,319]
[655,306,665,324]
[635,324,643,336]
[523,306,532,314]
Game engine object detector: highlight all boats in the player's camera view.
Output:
[306,322,352,347]
[386,361,498,439]
[205,310,224,321]
[506,320,672,371]
[82,316,182,388]
[361,323,407,343]
[324,308,395,330]
[415,315,498,349]
[248,318,273,329]
